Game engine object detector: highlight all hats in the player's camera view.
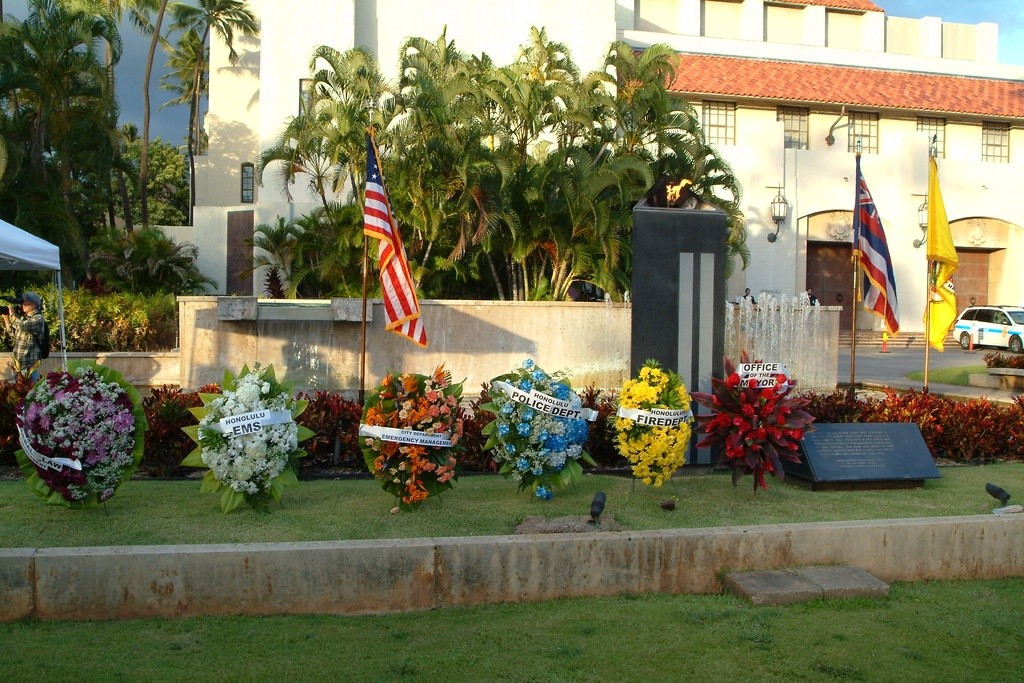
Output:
[23,292,41,305]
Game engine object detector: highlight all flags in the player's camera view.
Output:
[851,151,899,336]
[923,157,959,352]
[364,125,428,348]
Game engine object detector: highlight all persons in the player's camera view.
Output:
[743,288,756,304]
[2,292,45,381]
[807,288,817,306]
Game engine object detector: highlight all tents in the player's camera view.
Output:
[0,219,67,372]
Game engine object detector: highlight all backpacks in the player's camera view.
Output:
[32,313,50,359]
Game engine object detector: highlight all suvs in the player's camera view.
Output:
[952,305,1024,354]
